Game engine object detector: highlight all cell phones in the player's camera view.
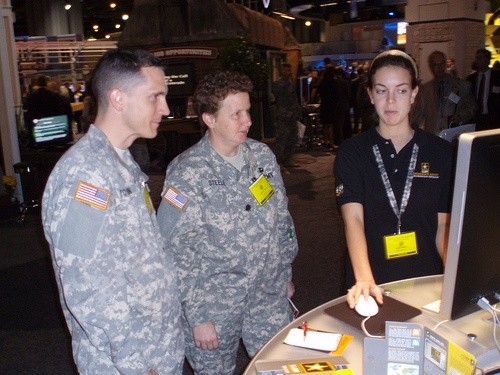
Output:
[288,298,299,318]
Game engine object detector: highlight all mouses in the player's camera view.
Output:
[354,294,379,316]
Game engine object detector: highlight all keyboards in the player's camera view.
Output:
[421,299,441,314]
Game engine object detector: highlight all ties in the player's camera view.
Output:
[437,79,447,118]
[477,73,485,114]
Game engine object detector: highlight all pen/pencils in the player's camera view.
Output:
[303,320,307,336]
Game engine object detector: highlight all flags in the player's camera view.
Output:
[73,181,112,211]
[162,186,189,210]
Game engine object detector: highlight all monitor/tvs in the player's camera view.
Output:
[440,129,500,322]
[31,114,71,147]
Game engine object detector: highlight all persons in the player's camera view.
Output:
[157,71,298,375]
[27,49,500,166]
[334,49,453,309]
[41,49,186,375]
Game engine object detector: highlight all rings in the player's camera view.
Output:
[348,290,352,293]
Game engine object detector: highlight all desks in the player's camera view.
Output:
[245,275,500,375]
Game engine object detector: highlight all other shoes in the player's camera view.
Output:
[284,163,300,168]
[321,143,339,151]
[352,128,357,134]
